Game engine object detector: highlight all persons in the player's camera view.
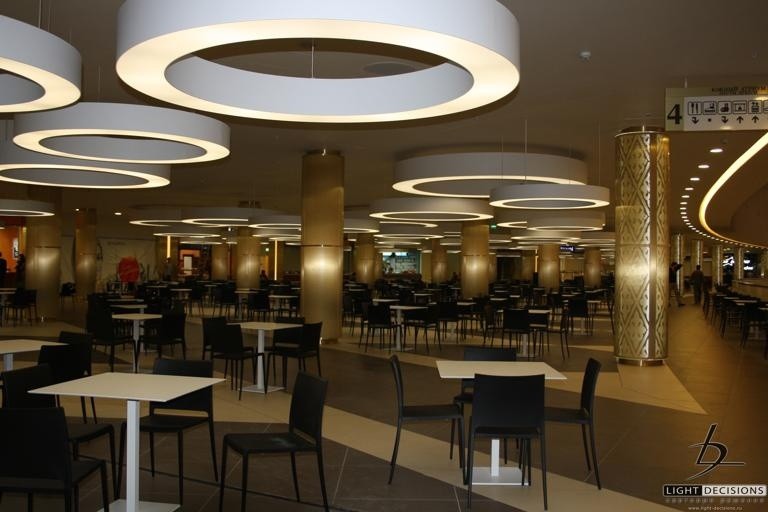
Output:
[0,252,6,288]
[260,270,268,287]
[289,270,300,284]
[669,262,686,307]
[15,254,25,288]
[375,267,461,296]
[165,258,172,282]
[690,265,704,304]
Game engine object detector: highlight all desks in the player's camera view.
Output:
[434,358,564,486]
[31,371,223,512]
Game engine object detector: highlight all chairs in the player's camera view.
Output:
[386,353,466,483]
[2,364,116,501]
[118,360,222,501]
[534,356,601,492]
[455,368,547,512]
[455,346,528,464]
[216,372,331,512]
[0,253,767,425]
[0,400,108,511]
